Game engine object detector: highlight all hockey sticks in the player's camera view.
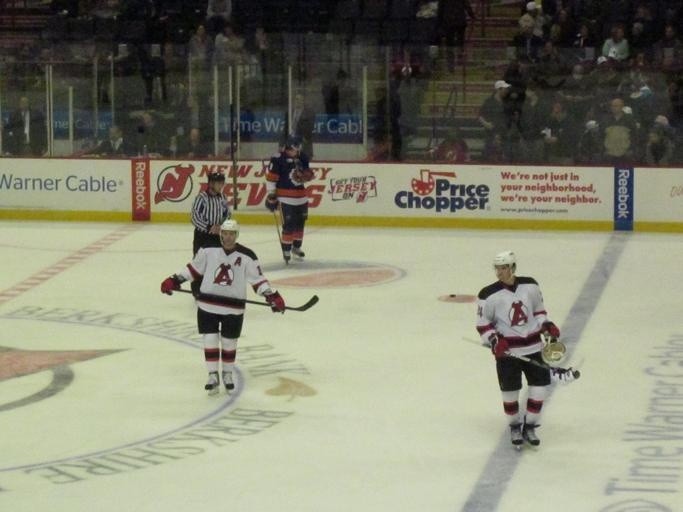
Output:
[465,337,580,385]
[173,289,318,311]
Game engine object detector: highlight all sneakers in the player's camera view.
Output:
[292,247,307,258]
[282,248,292,261]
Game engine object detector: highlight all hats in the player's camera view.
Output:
[493,80,512,92]
[540,48,672,144]
[527,2,541,11]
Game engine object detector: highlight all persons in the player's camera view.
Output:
[0,0,682,167]
[264,139,316,260]
[192,172,228,298]
[476,251,559,450]
[160,219,285,389]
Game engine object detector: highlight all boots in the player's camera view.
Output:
[223,371,235,391]
[509,422,523,445]
[523,416,541,446]
[204,372,220,391]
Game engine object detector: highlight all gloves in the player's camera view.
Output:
[264,289,287,315]
[302,167,315,182]
[160,273,184,297]
[490,333,511,360]
[541,320,561,340]
[264,194,279,213]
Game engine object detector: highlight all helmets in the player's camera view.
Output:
[207,171,226,183]
[219,218,240,239]
[492,251,519,275]
[541,342,571,367]
[284,137,303,150]
